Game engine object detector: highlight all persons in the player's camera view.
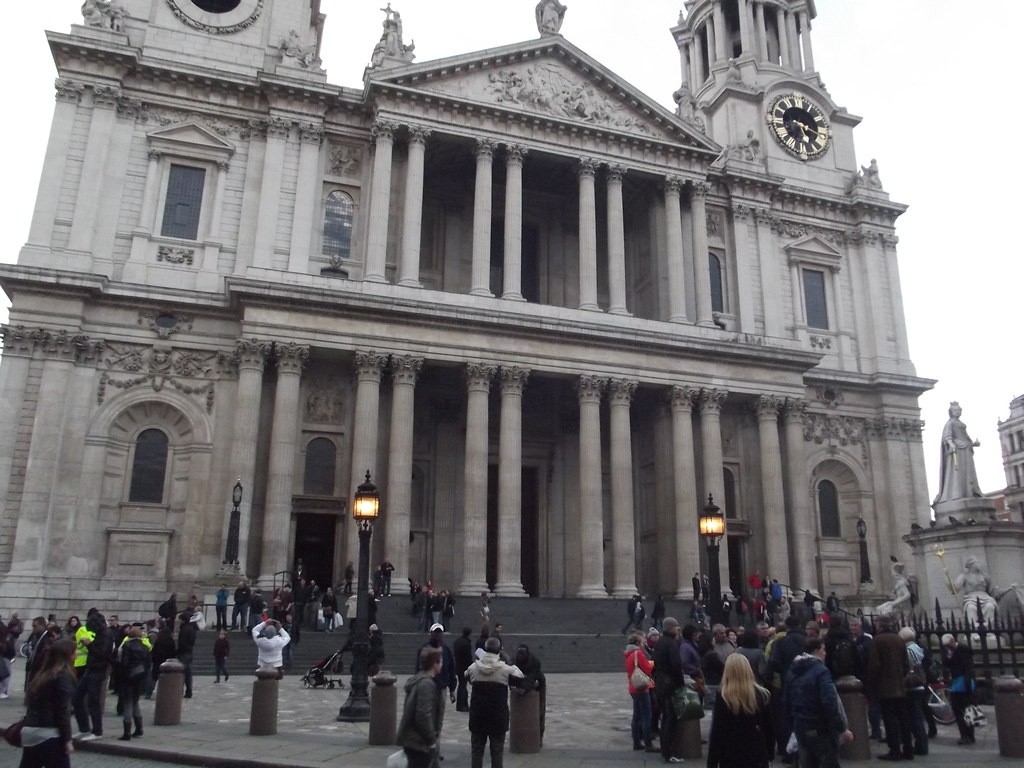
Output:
[535,0,567,37]
[282,28,312,62]
[0,559,546,768]
[372,10,415,65]
[939,400,984,504]
[81,0,127,31]
[946,559,1017,628]
[875,562,911,620]
[672,81,696,124]
[861,159,881,188]
[745,129,760,160]
[488,62,661,137]
[622,569,977,768]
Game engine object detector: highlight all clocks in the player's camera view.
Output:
[765,92,833,162]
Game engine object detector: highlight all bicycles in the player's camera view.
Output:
[927,682,957,724]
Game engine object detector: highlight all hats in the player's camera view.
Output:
[370,624,378,630]
[430,623,444,632]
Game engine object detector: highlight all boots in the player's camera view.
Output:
[131,716,144,737]
[118,721,131,740]
[644,738,661,752]
[632,735,645,750]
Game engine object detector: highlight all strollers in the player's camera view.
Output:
[300,645,349,690]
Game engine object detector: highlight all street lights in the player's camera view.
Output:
[336,469,379,722]
[698,492,726,632]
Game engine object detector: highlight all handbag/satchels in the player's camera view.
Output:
[671,686,705,720]
[904,647,926,689]
[5,722,21,748]
[631,667,650,689]
[964,704,988,727]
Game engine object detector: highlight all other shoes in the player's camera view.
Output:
[72,731,89,738]
[869,730,975,760]
[0,692,8,699]
[225,674,228,681]
[184,695,191,698]
[214,680,220,683]
[456,705,468,712]
[81,733,103,741]
